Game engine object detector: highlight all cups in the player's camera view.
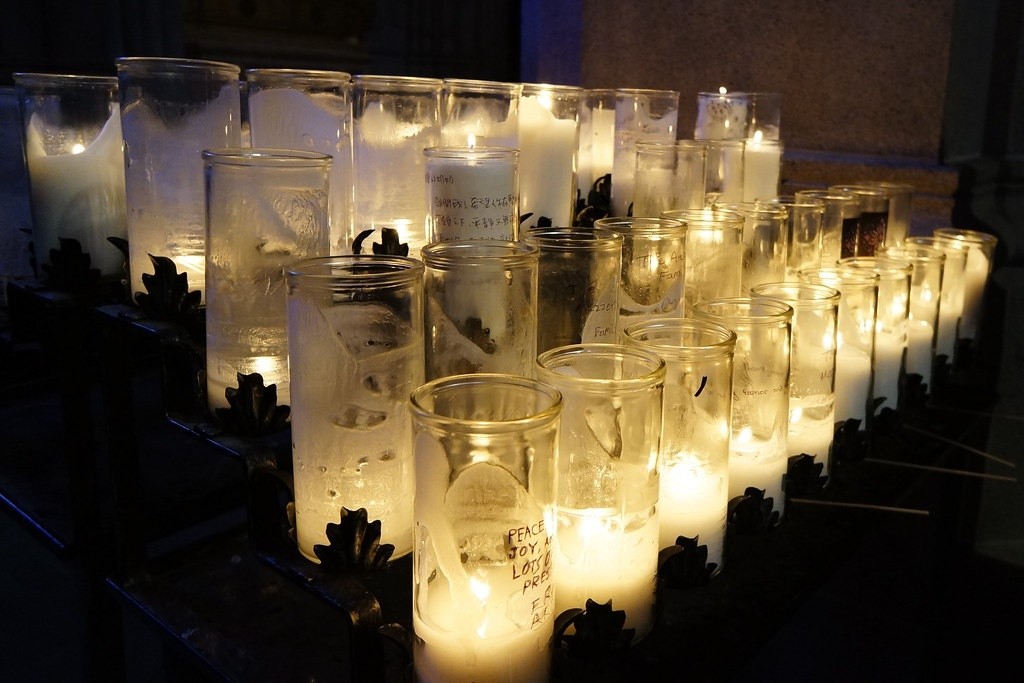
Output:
[243,67,354,261]
[408,373,552,683]
[199,147,335,422]
[728,203,788,290]
[878,243,946,393]
[838,255,915,412]
[423,145,519,249]
[606,88,679,217]
[692,89,746,197]
[658,207,743,314]
[622,315,732,583]
[677,137,746,210]
[12,73,128,289]
[752,281,838,489]
[829,182,892,254]
[115,59,244,312]
[421,239,541,382]
[578,84,619,198]
[934,228,996,342]
[283,254,412,566]
[798,269,881,432]
[722,137,786,204]
[692,295,788,522]
[906,234,973,365]
[347,74,443,260]
[754,194,825,276]
[533,343,660,649]
[517,226,625,360]
[793,190,861,264]
[858,182,914,247]
[634,142,707,218]
[516,82,584,234]
[439,78,519,161]
[594,218,684,321]
[727,92,783,143]
